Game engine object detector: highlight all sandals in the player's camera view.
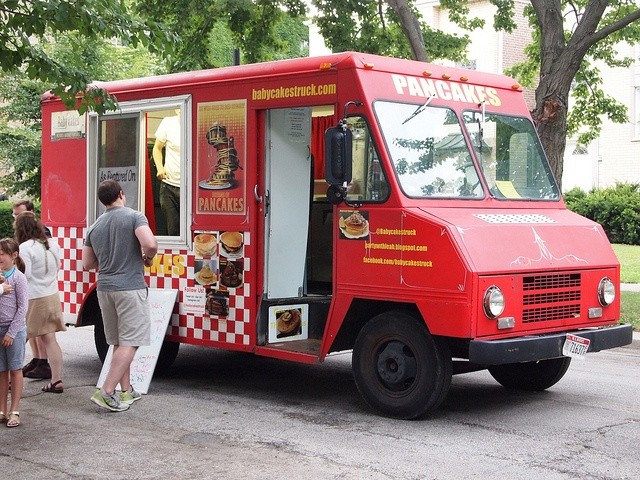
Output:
[41,380,64,393]
[6,411,20,427]
[0,411,9,423]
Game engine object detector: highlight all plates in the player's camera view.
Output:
[340,217,368,238]
[220,232,243,259]
[199,180,232,189]
[194,270,216,285]
[220,274,244,289]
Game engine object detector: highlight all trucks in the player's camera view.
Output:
[41,51,632,420]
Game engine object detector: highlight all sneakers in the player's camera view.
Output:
[119,384,142,405]
[21,363,37,374]
[89,387,130,412]
[26,365,52,378]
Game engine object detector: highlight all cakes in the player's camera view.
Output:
[344,214,368,235]
[198,264,214,283]
[206,122,242,186]
[220,261,241,287]
[277,309,301,335]
[208,292,227,315]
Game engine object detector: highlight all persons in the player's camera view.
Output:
[12,200,52,380]
[152,108,181,236]
[0,275,14,298]
[82,180,157,412]
[13,212,67,394]
[0,238,29,427]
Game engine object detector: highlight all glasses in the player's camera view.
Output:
[12,213,19,217]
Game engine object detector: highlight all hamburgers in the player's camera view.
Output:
[194,234,217,256]
[220,231,244,254]
